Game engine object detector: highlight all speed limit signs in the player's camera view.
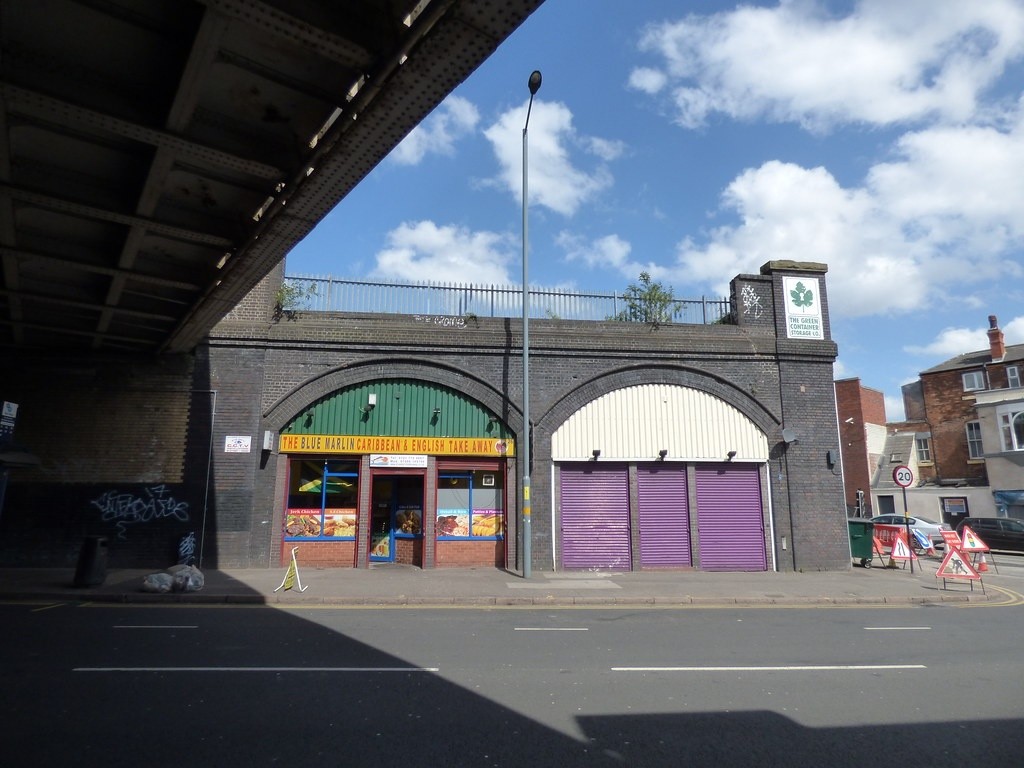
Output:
[894,465,913,488]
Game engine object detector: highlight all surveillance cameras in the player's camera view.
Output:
[368,394,377,409]
[845,417,853,423]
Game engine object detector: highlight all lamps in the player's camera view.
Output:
[489,415,500,421]
[660,450,668,459]
[727,451,737,460]
[307,411,314,416]
[433,408,441,413]
[365,405,372,411]
[592,450,601,459]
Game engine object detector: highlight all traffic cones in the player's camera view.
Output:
[942,542,951,558]
[927,535,935,554]
[976,551,987,571]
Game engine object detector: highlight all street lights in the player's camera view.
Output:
[520,70,543,580]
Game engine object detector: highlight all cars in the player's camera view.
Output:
[955,517,1024,552]
[869,514,952,544]
